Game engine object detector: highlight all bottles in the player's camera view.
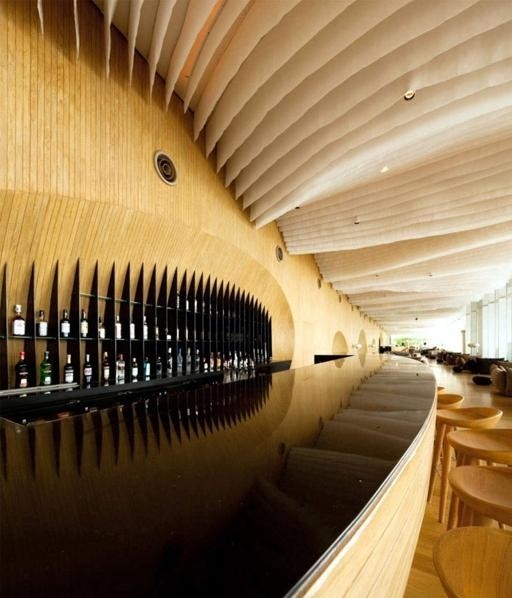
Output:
[194,345,194,348]
[165,345,253,376]
[11,302,164,389]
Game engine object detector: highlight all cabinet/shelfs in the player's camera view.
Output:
[0,256,274,399]
[0,368,273,482]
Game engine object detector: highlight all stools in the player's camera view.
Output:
[431,525,510,598]
[443,427,511,534]
[436,392,466,461]
[426,404,504,524]
[448,463,512,529]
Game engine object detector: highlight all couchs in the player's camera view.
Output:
[420,345,512,395]
